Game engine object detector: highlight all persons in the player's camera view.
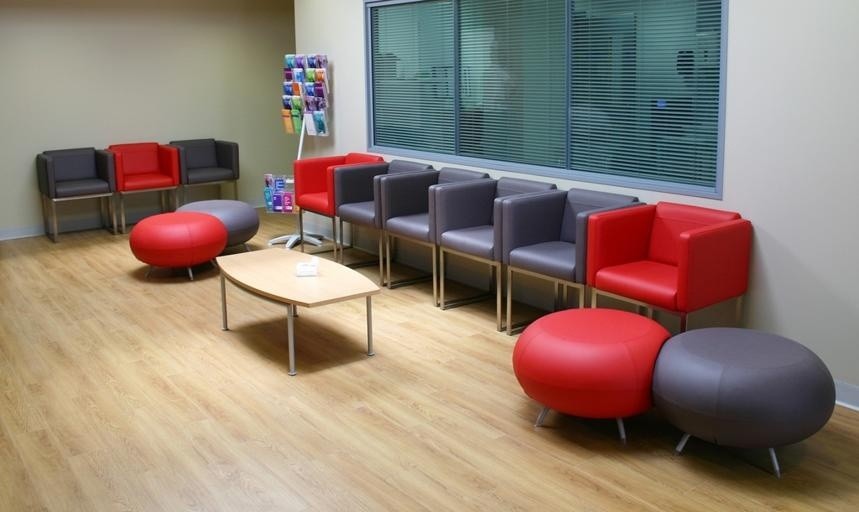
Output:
[482,40,516,156]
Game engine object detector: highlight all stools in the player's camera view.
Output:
[176,199,261,252]
[129,212,228,280]
[652,328,835,478]
[512,307,671,443]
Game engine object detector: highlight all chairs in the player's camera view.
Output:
[172,140,242,200]
[334,160,435,286]
[434,177,563,333]
[589,201,753,333]
[502,187,647,336]
[34,147,118,243]
[380,167,494,306]
[106,143,182,233]
[295,153,383,262]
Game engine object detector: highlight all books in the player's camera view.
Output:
[279,53,330,139]
[263,173,302,214]
